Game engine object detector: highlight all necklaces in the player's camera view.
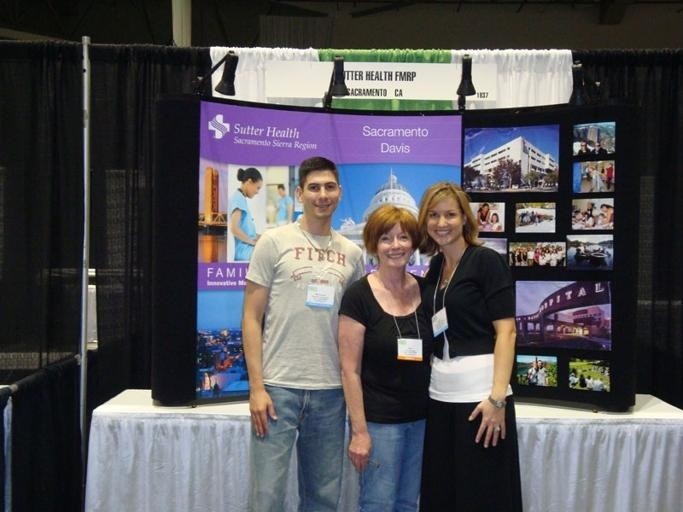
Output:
[441,259,453,285]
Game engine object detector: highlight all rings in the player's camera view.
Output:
[494,426,501,432]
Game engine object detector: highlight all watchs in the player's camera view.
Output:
[488,395,506,407]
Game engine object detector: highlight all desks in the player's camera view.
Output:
[84,384,683,512]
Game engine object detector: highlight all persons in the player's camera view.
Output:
[576,201,613,230]
[519,210,545,226]
[416,183,522,511]
[578,139,590,155]
[274,185,294,226]
[515,242,564,267]
[592,142,606,155]
[227,167,262,260]
[537,359,548,386]
[568,367,604,391]
[576,244,607,261]
[527,361,537,384]
[478,204,488,222]
[239,157,364,511]
[490,213,501,232]
[336,204,433,511]
